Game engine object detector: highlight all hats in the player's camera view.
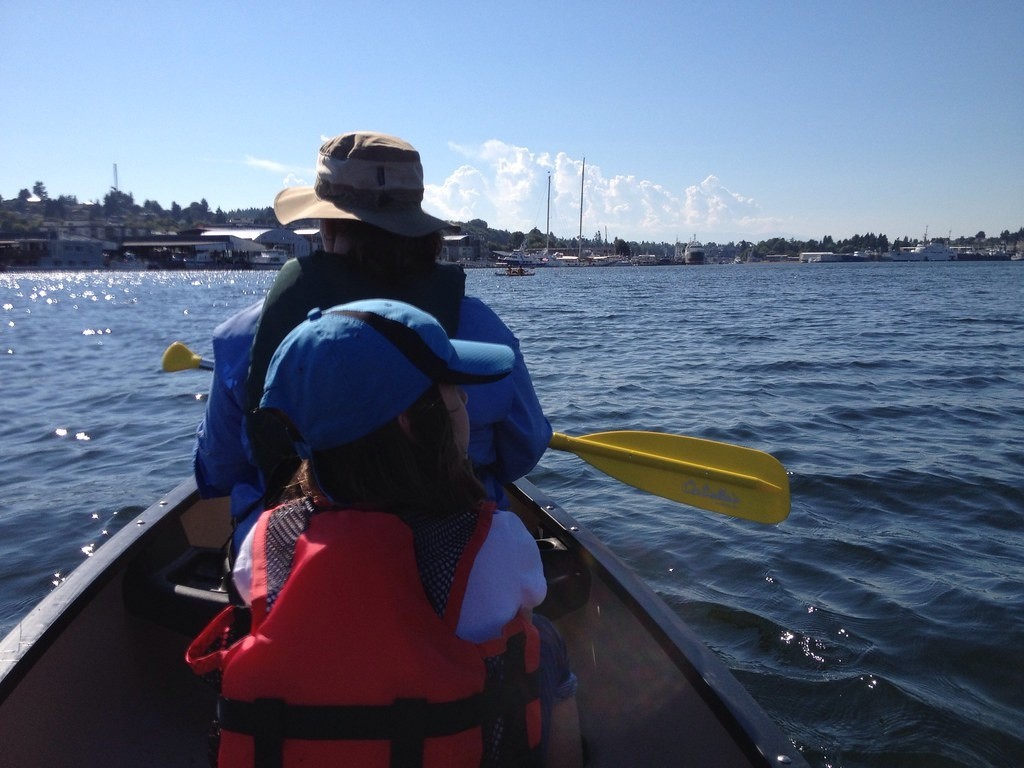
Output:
[274,130,462,236]
[260,297,514,462]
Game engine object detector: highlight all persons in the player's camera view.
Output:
[517,265,525,273]
[505,265,512,275]
[193,132,552,556]
[190,297,583,768]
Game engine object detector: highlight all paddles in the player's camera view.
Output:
[162,340,791,525]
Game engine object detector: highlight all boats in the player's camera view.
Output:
[638,255,658,266]
[888,239,958,262]
[684,232,705,265]
[494,269,535,277]
[249,249,289,271]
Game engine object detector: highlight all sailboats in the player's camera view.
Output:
[496,156,619,267]
